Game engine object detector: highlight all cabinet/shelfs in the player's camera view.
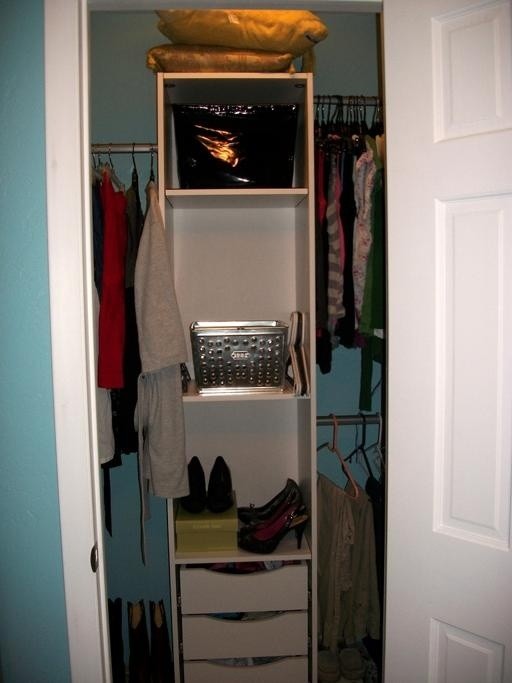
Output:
[158,72,317,682]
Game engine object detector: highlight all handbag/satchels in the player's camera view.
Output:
[190,320,287,397]
[174,102,298,188]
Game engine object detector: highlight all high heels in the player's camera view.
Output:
[237,478,308,554]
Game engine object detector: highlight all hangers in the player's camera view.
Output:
[91,143,159,192]
[356,412,385,500]
[316,413,358,500]
[342,413,379,504]
[312,95,385,154]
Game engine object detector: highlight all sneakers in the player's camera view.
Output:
[318,648,365,681]
[179,456,232,514]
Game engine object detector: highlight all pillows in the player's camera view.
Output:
[154,9,328,57]
[145,44,297,73]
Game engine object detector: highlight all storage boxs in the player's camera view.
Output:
[175,490,240,559]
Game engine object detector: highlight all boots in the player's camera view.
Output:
[107,598,171,683]
